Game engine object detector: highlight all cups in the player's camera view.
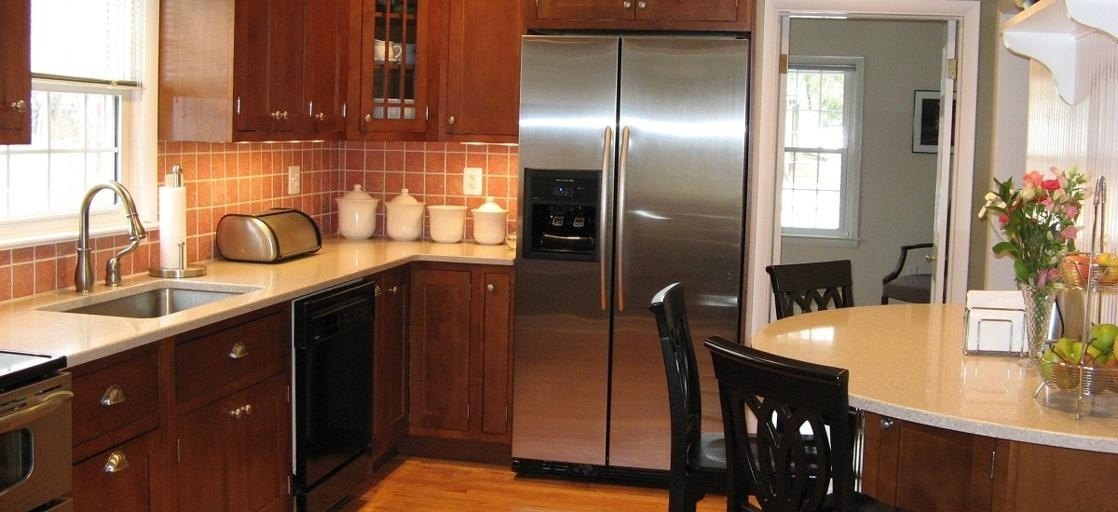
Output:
[374,39,401,62]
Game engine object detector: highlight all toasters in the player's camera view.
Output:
[215,208,323,263]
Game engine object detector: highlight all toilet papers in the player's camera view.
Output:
[155,170,189,271]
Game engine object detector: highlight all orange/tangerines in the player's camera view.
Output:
[1040,320,1118,393]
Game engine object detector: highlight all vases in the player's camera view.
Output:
[1017,281,1054,370]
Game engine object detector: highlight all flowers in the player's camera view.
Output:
[976,164,1096,362]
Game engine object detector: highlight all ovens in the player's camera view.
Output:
[0,373,75,512]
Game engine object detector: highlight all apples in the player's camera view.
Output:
[1092,253,1118,286]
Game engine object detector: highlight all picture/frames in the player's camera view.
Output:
[908,86,958,157]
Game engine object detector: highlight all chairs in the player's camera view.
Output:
[645,281,836,512]
[764,259,859,322]
[880,242,934,304]
[701,331,907,512]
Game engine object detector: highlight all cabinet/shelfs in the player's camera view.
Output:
[342,0,438,140]
[520,0,758,34]
[0,0,33,146]
[168,312,291,512]
[371,274,403,455]
[438,0,522,143]
[158,1,341,143]
[402,262,514,443]
[68,346,167,510]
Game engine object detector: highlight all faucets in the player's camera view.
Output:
[70,177,148,295]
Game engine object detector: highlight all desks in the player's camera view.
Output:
[747,300,1116,512]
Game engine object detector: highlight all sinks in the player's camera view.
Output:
[34,276,266,322]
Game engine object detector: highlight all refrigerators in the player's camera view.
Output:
[512,35,751,478]
